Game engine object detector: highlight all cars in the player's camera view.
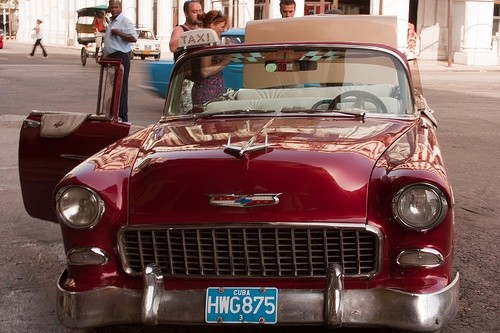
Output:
[129,27,162,61]
[148,28,321,99]
[18,14,460,333]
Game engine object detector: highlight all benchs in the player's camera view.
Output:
[207,84,405,115]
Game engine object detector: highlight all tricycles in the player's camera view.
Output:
[75,6,112,65]
